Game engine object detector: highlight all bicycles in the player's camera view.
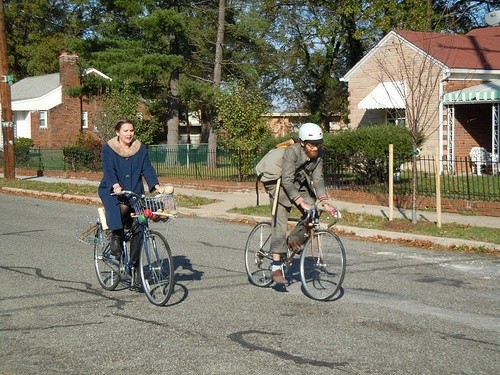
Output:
[94,190,178,307]
[244,206,347,302]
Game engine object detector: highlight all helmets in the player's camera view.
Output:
[298,122,324,141]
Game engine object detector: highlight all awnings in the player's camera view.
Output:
[357,80,408,110]
[444,82,500,105]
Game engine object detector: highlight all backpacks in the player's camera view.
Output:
[255,145,287,207]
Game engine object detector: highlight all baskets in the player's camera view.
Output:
[133,194,178,223]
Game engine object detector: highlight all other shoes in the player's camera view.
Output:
[287,236,302,254]
[271,270,288,284]
[110,234,122,253]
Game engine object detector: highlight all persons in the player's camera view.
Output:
[98,119,165,278]
[264,123,336,284]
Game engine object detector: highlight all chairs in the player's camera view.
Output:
[469,146,492,175]
